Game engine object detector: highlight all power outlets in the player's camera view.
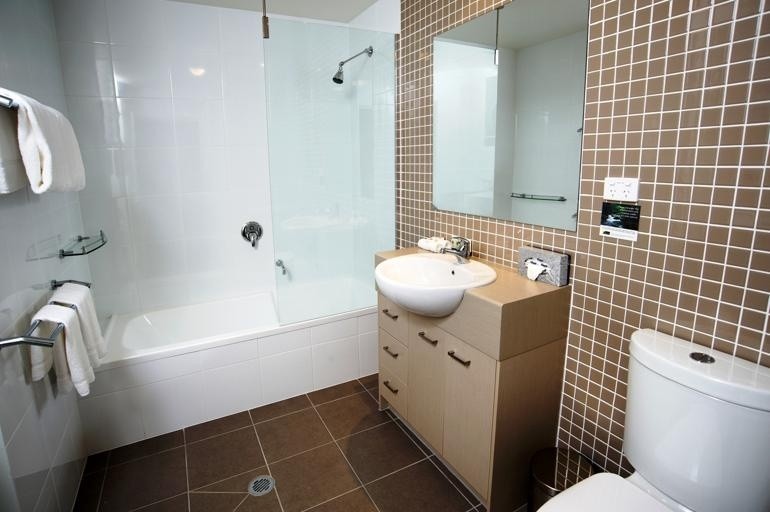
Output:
[603,178,639,202]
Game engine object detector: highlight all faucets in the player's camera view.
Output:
[241,222,264,250]
[440,237,472,258]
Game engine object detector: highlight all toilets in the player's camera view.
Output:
[531,326,770,512]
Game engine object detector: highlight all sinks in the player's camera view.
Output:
[374,252,497,317]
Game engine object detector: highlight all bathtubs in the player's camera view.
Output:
[76,274,379,455]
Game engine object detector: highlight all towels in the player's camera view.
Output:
[29,281,109,396]
[0,89,87,195]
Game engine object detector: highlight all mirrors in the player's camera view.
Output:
[431,1,590,233]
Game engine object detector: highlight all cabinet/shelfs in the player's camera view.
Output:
[377,293,501,512]
